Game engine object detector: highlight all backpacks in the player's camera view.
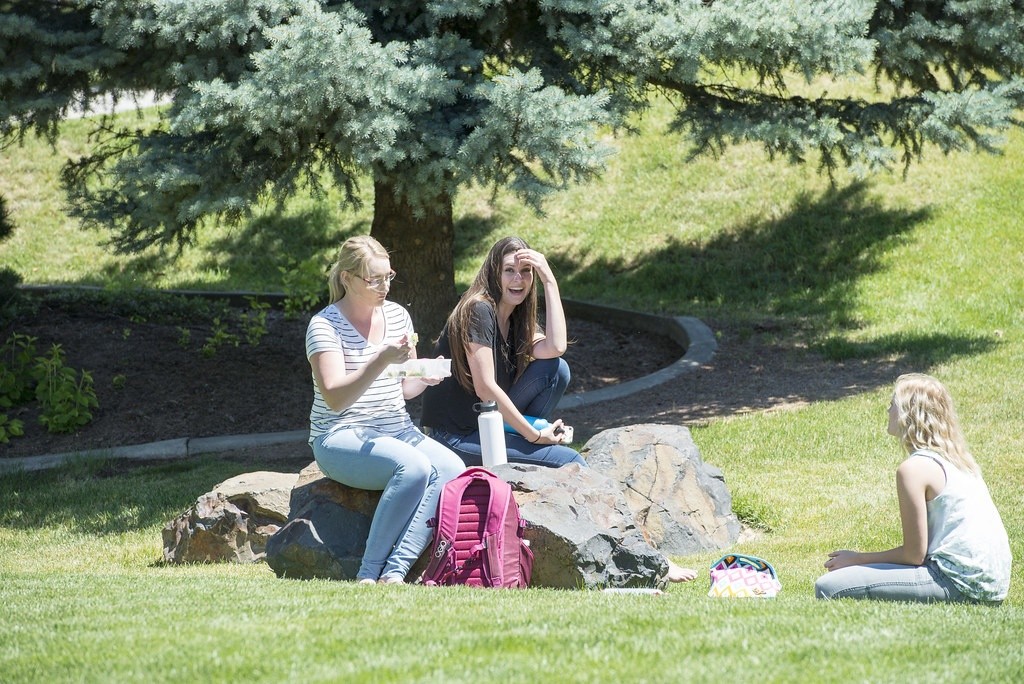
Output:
[421,468,536,590]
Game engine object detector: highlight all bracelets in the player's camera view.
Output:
[528,430,541,444]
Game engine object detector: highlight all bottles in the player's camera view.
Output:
[523,540,530,548]
[472,400,507,467]
[504,415,564,433]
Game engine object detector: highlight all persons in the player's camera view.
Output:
[815,374,1015,604]
[305,233,469,584]
[419,235,589,469]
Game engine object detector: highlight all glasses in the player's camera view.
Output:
[348,269,396,286]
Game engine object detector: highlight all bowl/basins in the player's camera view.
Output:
[392,358,452,378]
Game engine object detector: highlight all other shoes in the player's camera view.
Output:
[357,576,405,586]
